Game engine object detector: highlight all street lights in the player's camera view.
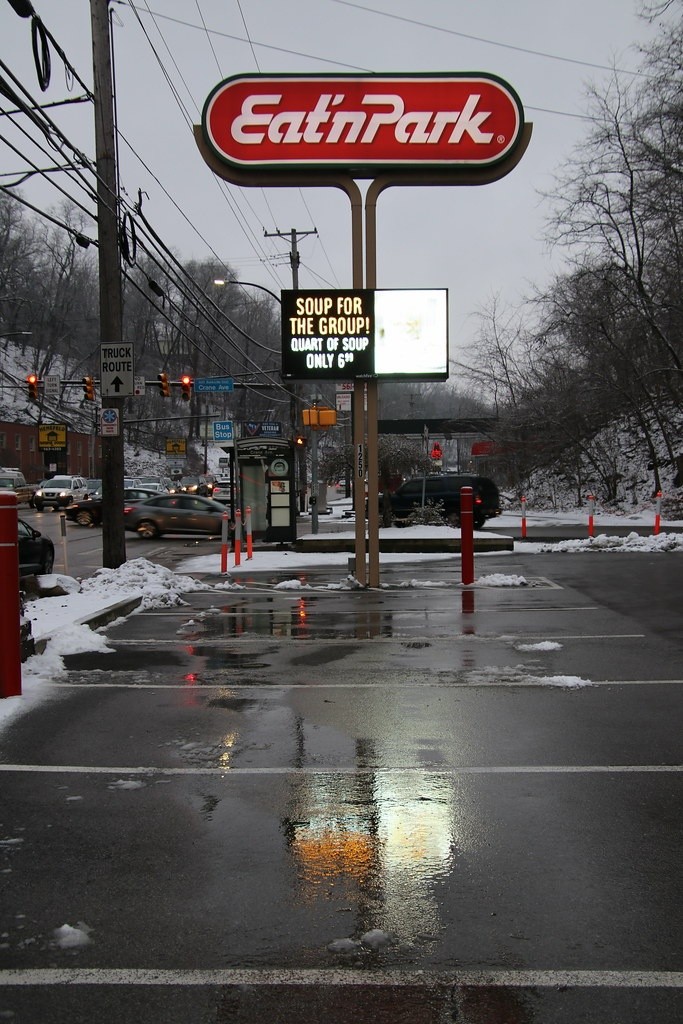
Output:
[214,279,281,306]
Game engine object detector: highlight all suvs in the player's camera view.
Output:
[365,472,501,529]
[34,475,88,512]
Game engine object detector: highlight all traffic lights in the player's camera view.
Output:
[182,376,191,402]
[158,373,170,397]
[296,437,305,446]
[82,376,94,401]
[28,375,38,400]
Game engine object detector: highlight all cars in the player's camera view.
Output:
[124,474,231,498]
[212,480,241,504]
[18,518,55,577]
[336,479,353,493]
[86,479,102,500]
[64,488,166,526]
[124,494,234,540]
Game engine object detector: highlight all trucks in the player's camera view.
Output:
[0,468,39,509]
